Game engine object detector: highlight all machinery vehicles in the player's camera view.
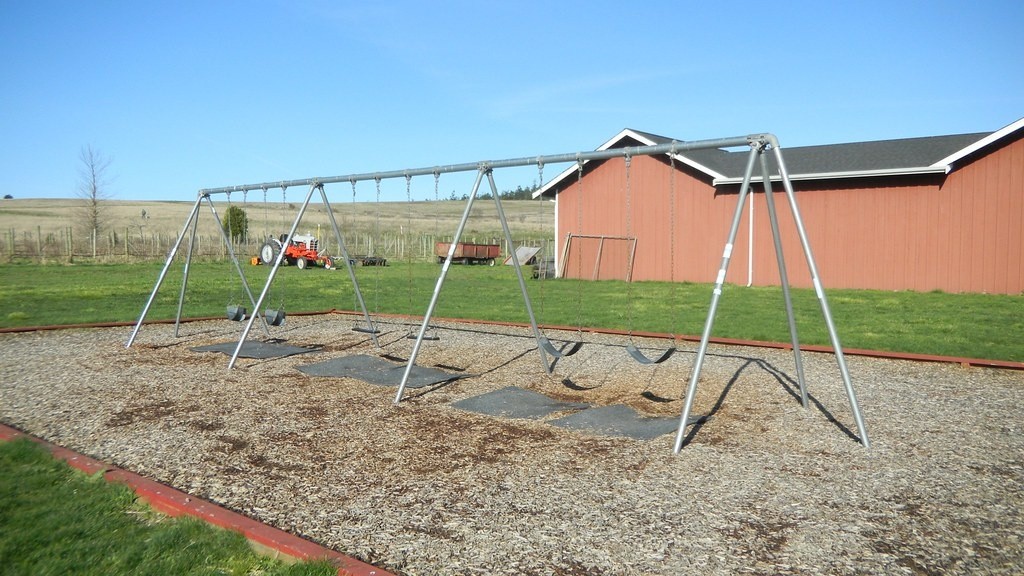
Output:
[260,224,334,270]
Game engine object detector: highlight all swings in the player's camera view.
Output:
[351,186,382,334]
[227,195,246,322]
[406,180,440,340]
[263,192,286,326]
[626,153,679,366]
[538,168,584,359]
